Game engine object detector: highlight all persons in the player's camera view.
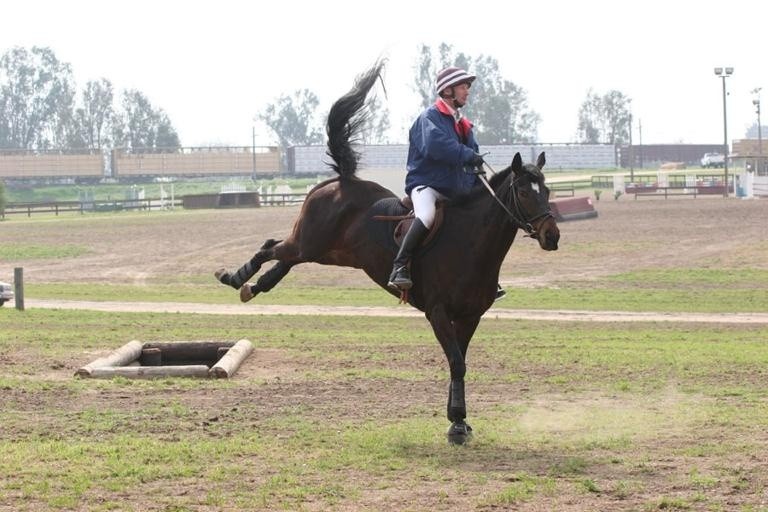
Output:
[385,66,508,299]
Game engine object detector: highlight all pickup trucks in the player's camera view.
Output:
[700,152,724,167]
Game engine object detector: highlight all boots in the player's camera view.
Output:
[387,216,430,293]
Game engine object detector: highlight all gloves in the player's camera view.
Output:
[466,152,484,167]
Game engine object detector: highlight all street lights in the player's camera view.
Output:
[714,67,734,194]
[751,86,763,139]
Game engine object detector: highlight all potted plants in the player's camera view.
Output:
[594,189,602,200]
[613,191,622,200]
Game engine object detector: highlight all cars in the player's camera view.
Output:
[0,283,13,305]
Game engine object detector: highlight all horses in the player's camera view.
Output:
[208,54,563,445]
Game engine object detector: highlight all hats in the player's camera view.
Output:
[435,66,476,94]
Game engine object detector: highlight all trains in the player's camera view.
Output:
[1,140,767,181]
